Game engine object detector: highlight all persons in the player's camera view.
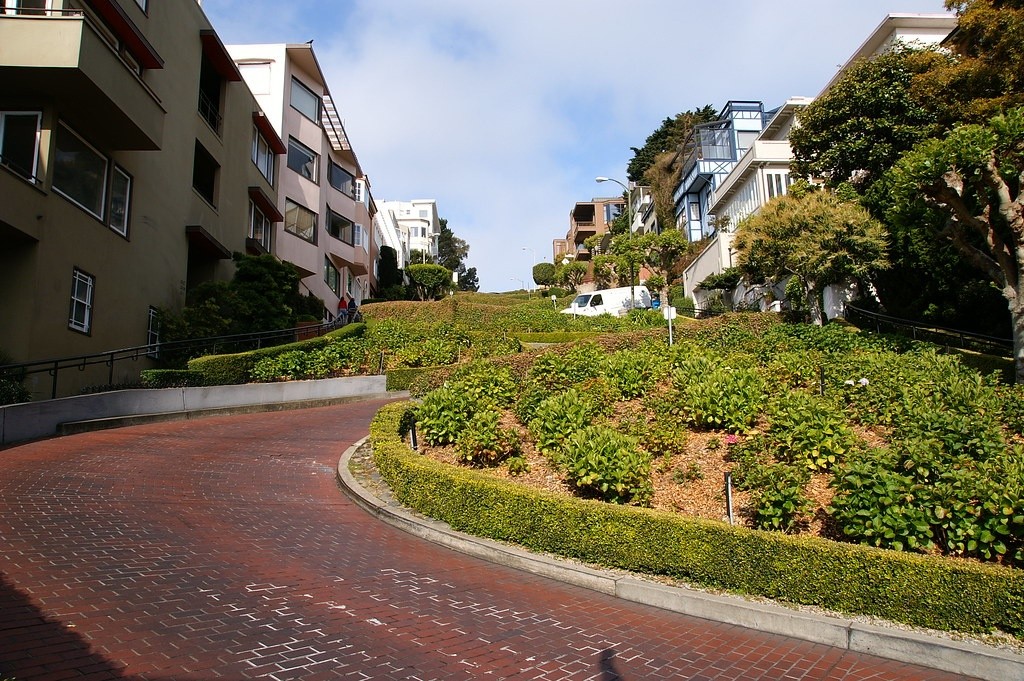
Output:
[338,296,347,322]
[348,298,355,309]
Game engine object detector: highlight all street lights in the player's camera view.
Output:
[596,176,634,310]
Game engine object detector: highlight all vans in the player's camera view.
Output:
[559,285,652,319]
